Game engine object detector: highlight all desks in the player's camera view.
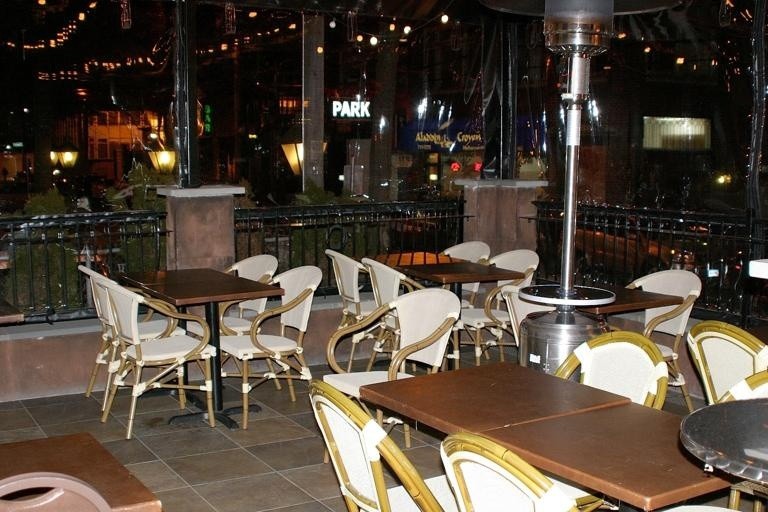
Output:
[1,292,25,328]
[0,430,163,512]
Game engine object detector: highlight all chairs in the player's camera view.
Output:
[1,469,112,512]
